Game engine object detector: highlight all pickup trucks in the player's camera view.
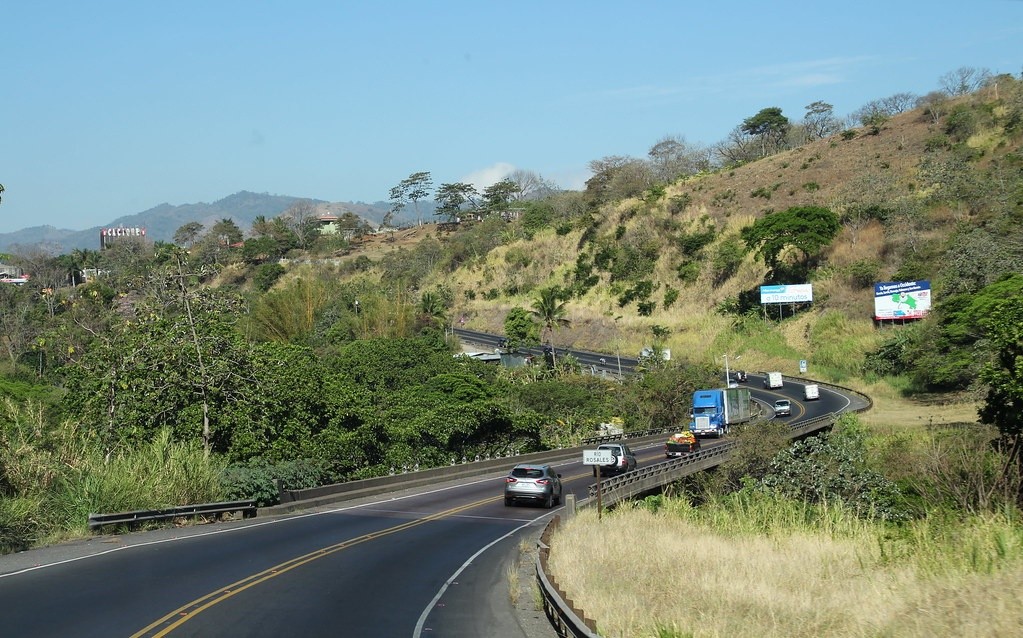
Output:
[664,432,701,457]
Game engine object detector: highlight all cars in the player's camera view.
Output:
[497,338,511,347]
[504,462,564,509]
[592,443,638,477]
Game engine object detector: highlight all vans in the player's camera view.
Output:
[773,399,792,416]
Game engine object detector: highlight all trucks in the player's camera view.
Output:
[763,372,783,390]
[803,384,820,400]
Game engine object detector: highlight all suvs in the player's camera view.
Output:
[732,370,748,383]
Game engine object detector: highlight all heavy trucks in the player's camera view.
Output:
[689,387,752,438]
